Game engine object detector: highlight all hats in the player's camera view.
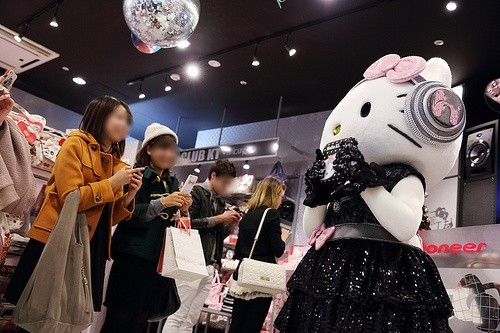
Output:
[141,122,178,149]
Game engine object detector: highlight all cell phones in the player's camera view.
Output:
[140,166,149,174]
[0,69,17,91]
[180,175,198,195]
[237,211,242,213]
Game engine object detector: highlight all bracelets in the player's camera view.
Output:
[160,197,167,207]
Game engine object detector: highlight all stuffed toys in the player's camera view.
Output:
[275,54,473,333]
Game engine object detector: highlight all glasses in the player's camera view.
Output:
[277,193,285,201]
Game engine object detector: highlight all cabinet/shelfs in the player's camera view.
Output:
[0,101,67,293]
[218,192,296,277]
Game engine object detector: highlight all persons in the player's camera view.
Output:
[0,70,290,333]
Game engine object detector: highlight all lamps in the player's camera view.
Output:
[14,25,28,42]
[137,83,145,98]
[251,44,260,66]
[194,165,201,174]
[163,75,171,91]
[283,34,297,57]
[50,4,58,27]
[242,160,249,168]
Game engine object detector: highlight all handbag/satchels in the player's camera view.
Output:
[266,160,287,185]
[203,268,223,311]
[157,207,209,281]
[12,186,95,333]
[237,257,286,294]
